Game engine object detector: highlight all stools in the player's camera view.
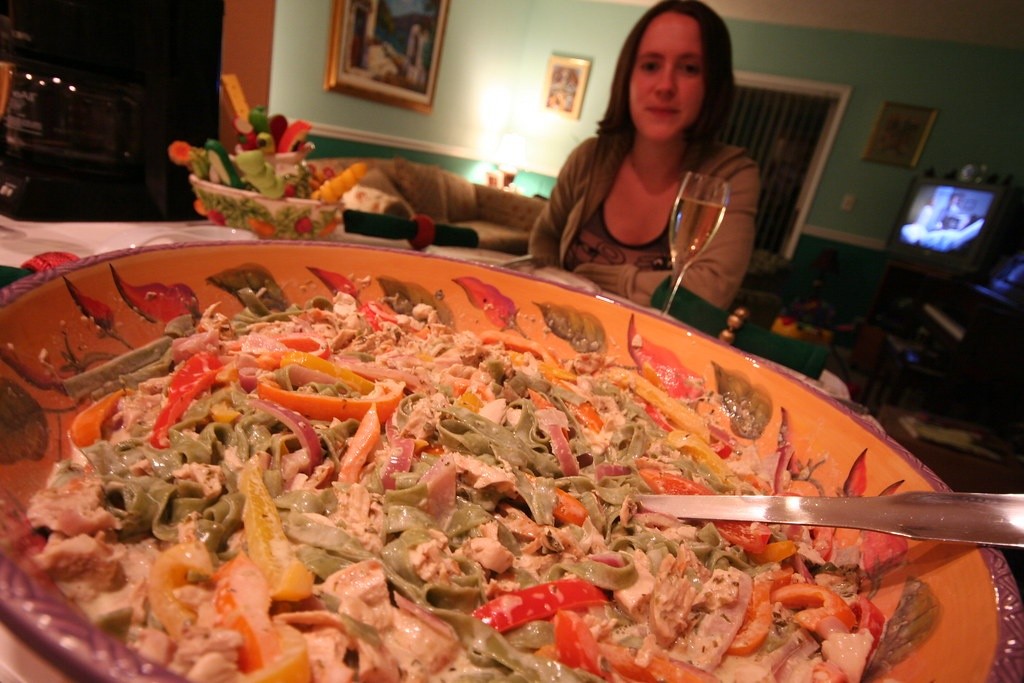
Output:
[860,334,970,408]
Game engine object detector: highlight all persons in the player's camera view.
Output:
[529,0,760,311]
[935,193,960,230]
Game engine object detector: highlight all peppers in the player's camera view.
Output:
[68,304,890,683]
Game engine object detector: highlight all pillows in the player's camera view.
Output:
[394,156,447,225]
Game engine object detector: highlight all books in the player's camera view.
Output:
[901,415,1000,460]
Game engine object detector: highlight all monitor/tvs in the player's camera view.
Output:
[883,175,1012,275]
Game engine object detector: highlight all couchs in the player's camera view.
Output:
[302,157,547,255]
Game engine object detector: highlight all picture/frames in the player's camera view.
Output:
[323,0,450,116]
[545,52,593,120]
[862,100,941,169]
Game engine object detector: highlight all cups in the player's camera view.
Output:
[659,171,732,317]
[168,227,258,242]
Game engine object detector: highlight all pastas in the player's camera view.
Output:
[65,297,791,683]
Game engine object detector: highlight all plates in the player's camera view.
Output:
[425,243,603,299]
[0,242,1024,683]
